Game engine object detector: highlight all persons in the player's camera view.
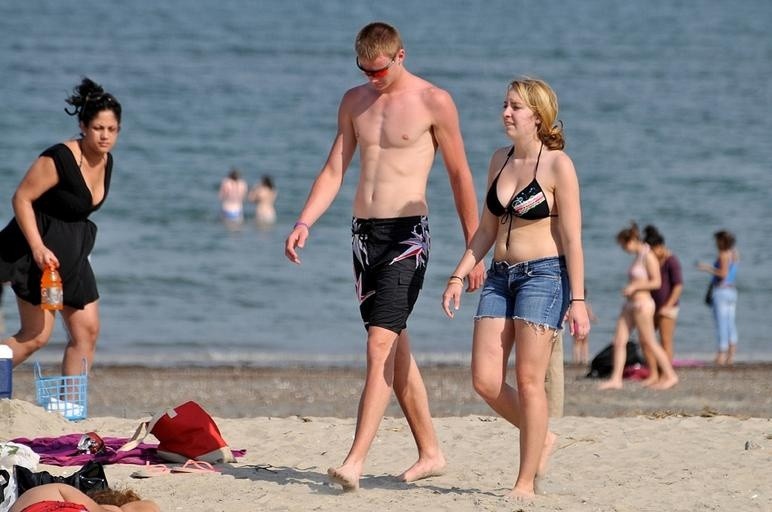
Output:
[284,24,487,491]
[696,232,739,365]
[8,482,159,512]
[596,223,678,391]
[442,80,591,501]
[573,288,596,365]
[248,174,276,227]
[638,225,682,387]
[0,78,122,402]
[218,168,248,229]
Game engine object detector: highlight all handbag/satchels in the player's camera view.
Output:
[13,460,109,498]
[116,400,236,465]
[705,281,720,304]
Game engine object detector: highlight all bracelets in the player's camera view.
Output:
[294,223,309,230]
[569,299,585,304]
[448,280,464,287]
[450,276,462,281]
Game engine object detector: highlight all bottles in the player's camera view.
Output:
[39,261,66,310]
[0,344,14,400]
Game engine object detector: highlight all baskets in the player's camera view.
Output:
[34,357,88,421]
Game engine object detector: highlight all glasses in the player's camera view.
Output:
[85,92,119,103]
[356,52,395,77]
